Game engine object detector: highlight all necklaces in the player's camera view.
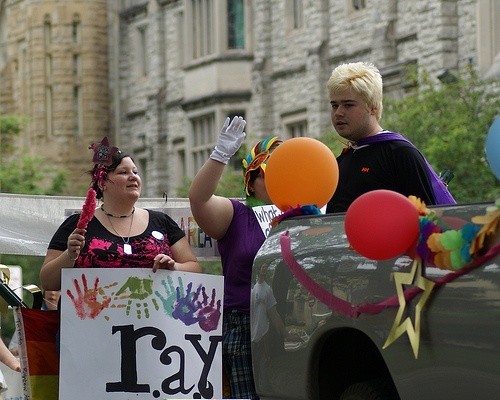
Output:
[101,204,135,255]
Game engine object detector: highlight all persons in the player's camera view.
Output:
[39,136,202,291]
[188,116,284,400]
[327,61,457,213]
[7,291,60,358]
[250,263,284,394]
[0,338,22,400]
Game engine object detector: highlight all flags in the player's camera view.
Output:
[13,306,60,400]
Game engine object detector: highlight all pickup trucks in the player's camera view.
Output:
[250,203,500,400]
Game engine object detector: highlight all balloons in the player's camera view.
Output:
[485,116,500,180]
[264,137,340,212]
[344,189,419,259]
